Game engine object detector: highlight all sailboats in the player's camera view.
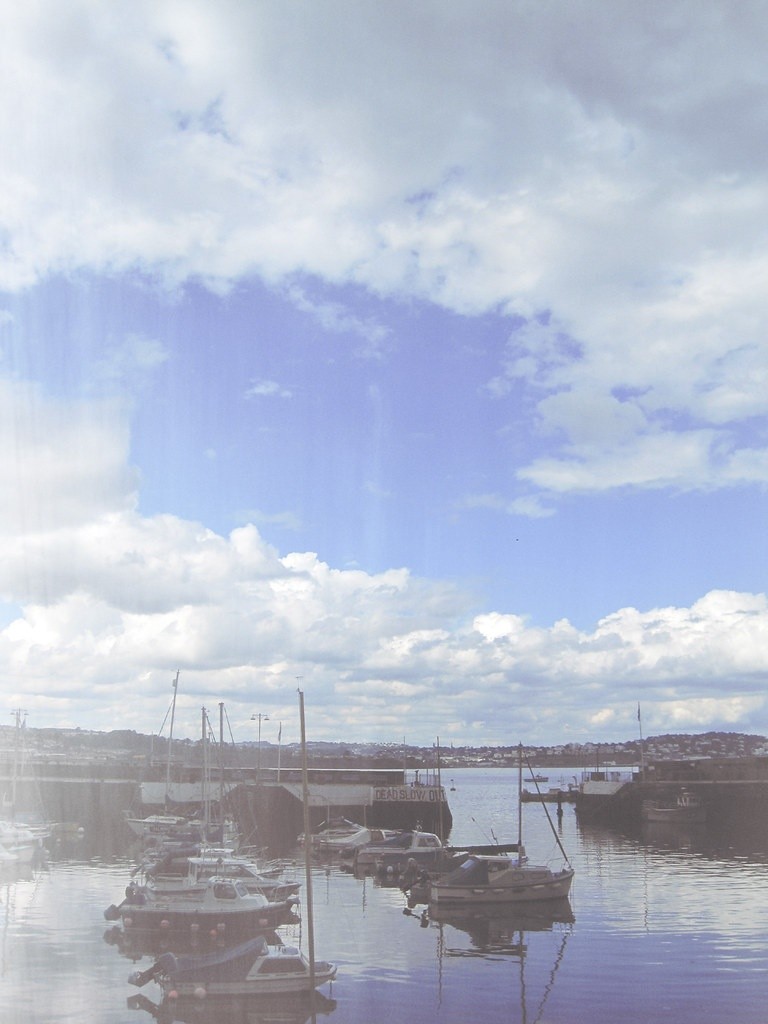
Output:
[0,700,302,896]
[428,734,575,905]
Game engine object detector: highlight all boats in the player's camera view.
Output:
[101,878,300,934]
[406,872,446,910]
[297,816,371,856]
[641,785,705,822]
[370,829,402,843]
[131,936,339,998]
[352,828,520,874]
[523,777,549,783]
[520,786,582,801]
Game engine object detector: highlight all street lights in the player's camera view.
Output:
[250,712,271,786]
